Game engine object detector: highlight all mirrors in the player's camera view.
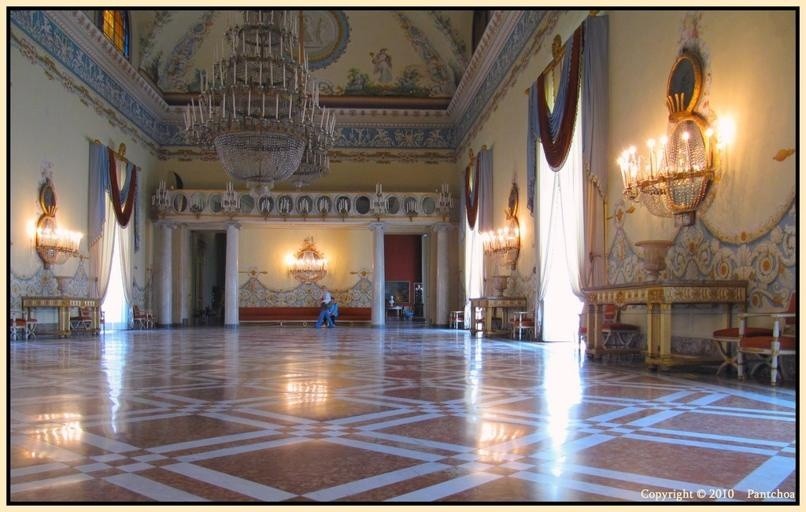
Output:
[659,115,713,226]
[35,214,61,265]
[503,218,520,262]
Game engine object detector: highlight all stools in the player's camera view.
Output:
[510,319,532,339]
[28,319,37,338]
[70,317,92,336]
[601,324,640,358]
[449,311,464,329]
[711,328,783,380]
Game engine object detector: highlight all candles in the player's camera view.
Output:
[289,259,327,271]
[481,228,519,253]
[37,231,82,251]
[618,134,710,189]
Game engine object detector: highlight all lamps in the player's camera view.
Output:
[285,148,331,189]
[183,10,336,197]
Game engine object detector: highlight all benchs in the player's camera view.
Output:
[239,307,371,328]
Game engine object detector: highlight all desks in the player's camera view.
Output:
[581,280,749,372]
[469,296,527,338]
[22,296,100,339]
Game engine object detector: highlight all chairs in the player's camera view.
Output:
[737,291,797,387]
[513,312,534,341]
[133,305,153,329]
[79,308,105,331]
[10,311,28,341]
[579,304,618,348]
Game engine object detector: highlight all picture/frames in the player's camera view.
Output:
[386,281,410,305]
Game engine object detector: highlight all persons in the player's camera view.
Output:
[327,297,338,327]
[316,284,335,329]
